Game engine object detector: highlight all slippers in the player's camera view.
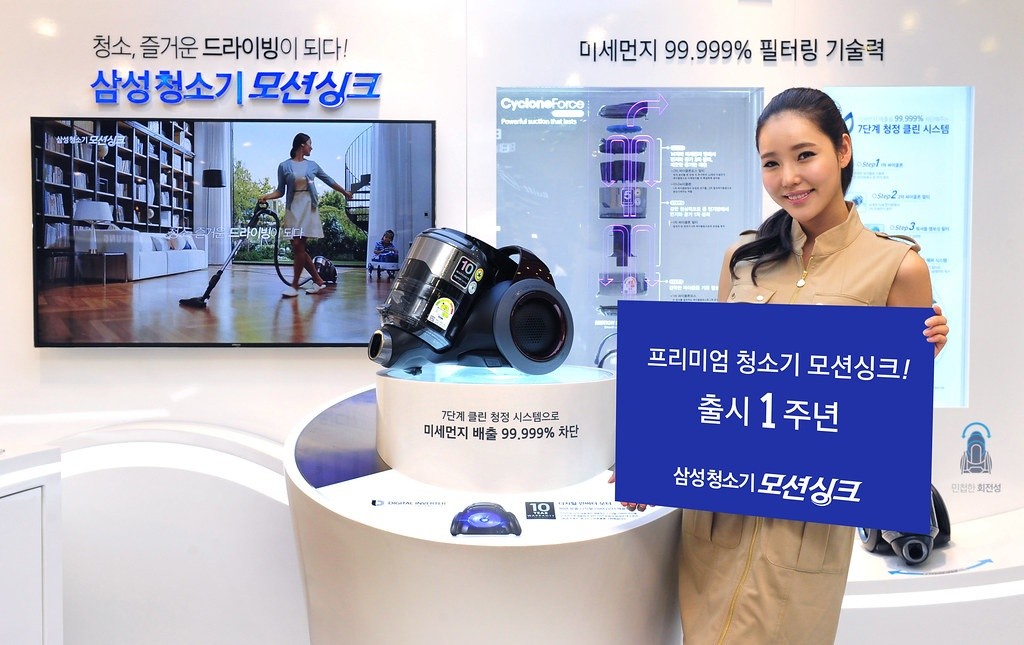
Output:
[282,287,299,296]
[305,283,326,294]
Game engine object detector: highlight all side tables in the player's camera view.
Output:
[74,252,128,286]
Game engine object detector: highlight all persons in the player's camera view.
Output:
[373,229,397,278]
[258,132,352,297]
[610,89,949,645]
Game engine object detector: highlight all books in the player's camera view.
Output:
[44,120,194,282]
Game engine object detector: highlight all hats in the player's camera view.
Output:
[382,229,394,242]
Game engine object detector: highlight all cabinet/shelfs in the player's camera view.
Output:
[31,117,194,285]
[0,444,62,645]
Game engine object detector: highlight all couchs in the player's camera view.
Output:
[75,229,208,280]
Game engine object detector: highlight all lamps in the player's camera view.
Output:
[73,200,114,254]
[202,169,227,234]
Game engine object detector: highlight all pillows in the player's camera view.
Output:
[149,230,197,251]
